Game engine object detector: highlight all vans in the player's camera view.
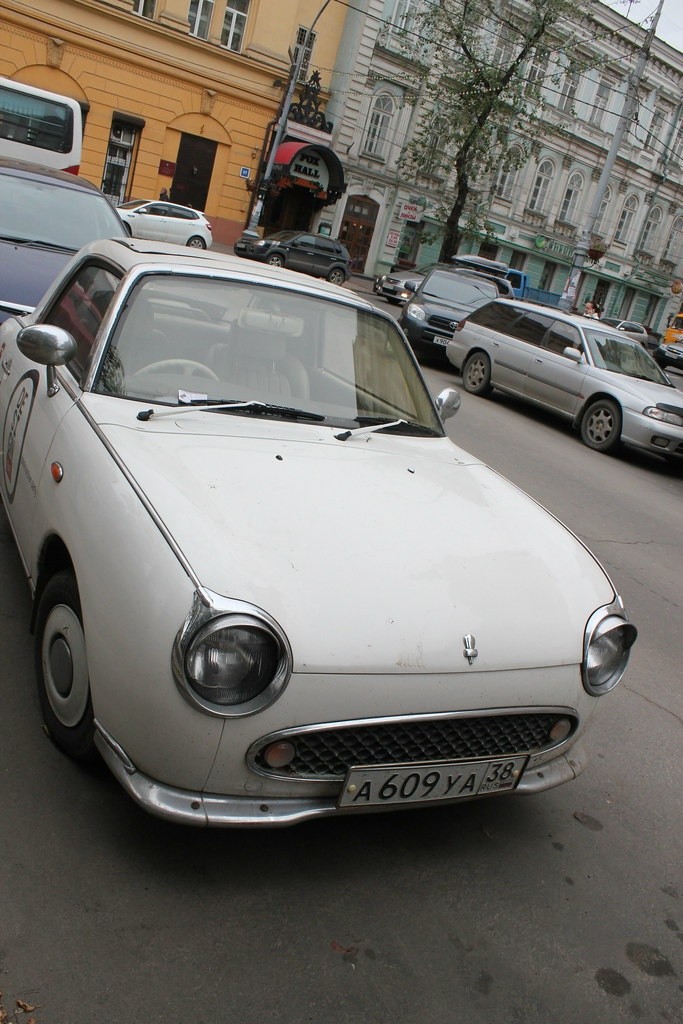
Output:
[0,76,82,176]
[375,262,515,305]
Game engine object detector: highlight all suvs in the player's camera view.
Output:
[234,229,353,286]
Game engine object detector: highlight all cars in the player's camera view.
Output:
[600,318,648,350]
[0,153,133,326]
[115,199,213,250]
[445,297,683,461]
[396,266,499,360]
[0,236,638,831]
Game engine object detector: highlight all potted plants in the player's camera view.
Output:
[588,242,605,260]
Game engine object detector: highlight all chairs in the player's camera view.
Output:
[203,319,311,400]
[113,297,200,381]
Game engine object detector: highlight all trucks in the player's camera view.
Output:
[504,269,562,306]
[654,303,683,370]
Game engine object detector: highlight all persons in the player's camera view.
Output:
[158,187,169,202]
[582,301,601,320]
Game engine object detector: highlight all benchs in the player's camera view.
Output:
[103,308,232,366]
